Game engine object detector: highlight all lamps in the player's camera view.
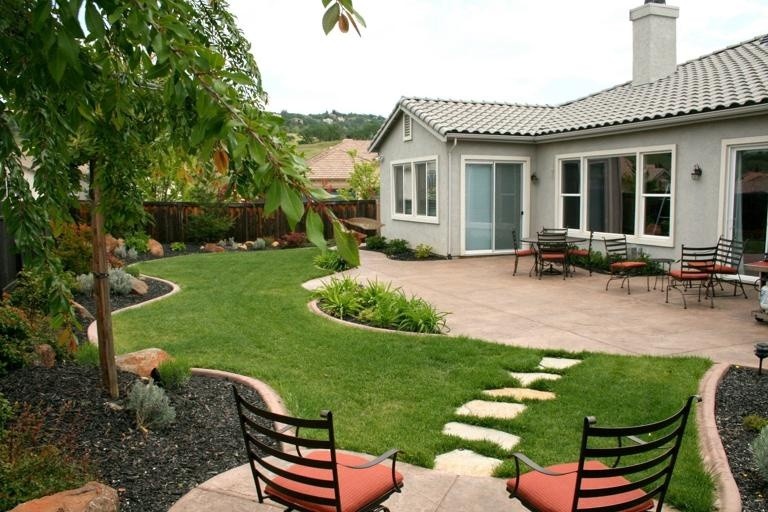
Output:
[690,163,701,179]
[531,172,538,186]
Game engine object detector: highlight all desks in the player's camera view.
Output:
[744,261,768,300]
[650,258,675,292]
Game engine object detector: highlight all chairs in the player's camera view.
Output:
[604,234,650,295]
[506,395,703,512]
[231,384,404,512]
[512,226,593,281]
[665,244,719,309]
[684,236,749,300]
